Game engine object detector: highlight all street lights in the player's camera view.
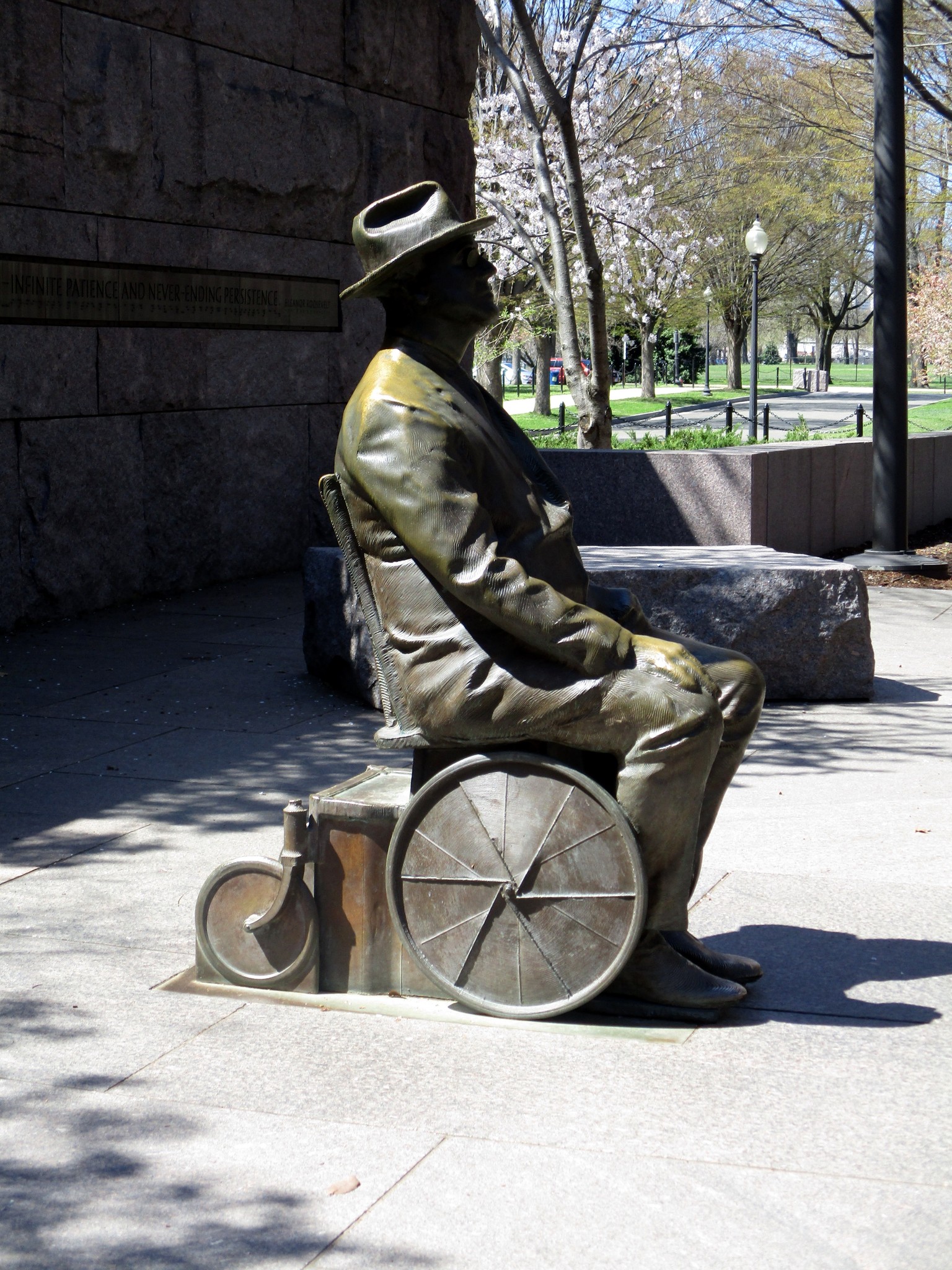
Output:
[702,285,714,396]
[744,213,769,440]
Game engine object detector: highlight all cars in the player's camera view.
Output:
[472,361,531,385]
[549,357,588,385]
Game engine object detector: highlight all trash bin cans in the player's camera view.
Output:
[550,372,559,385]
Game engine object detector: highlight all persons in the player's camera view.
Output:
[334,178,766,1011]
